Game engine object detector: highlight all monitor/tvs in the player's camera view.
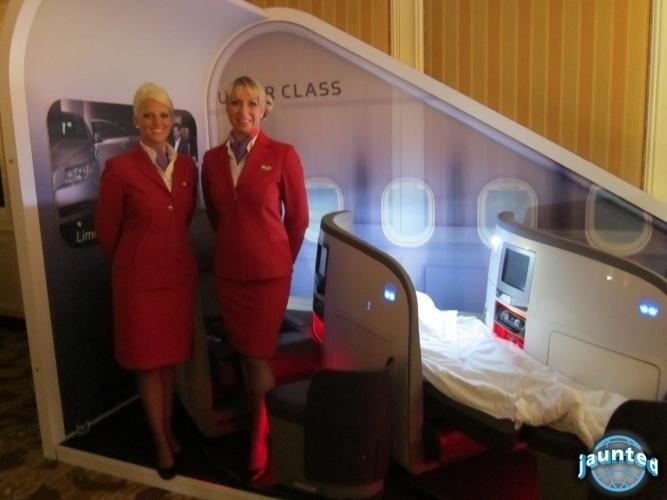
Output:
[496,241,536,305]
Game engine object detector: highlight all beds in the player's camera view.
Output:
[415,291,629,462]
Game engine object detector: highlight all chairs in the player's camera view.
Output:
[593,391,666,500]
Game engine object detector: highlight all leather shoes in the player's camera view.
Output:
[158,462,175,480]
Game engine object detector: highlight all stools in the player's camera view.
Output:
[266,376,393,499]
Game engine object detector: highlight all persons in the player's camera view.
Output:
[170,122,189,155]
[95,82,199,473]
[200,75,310,480]
